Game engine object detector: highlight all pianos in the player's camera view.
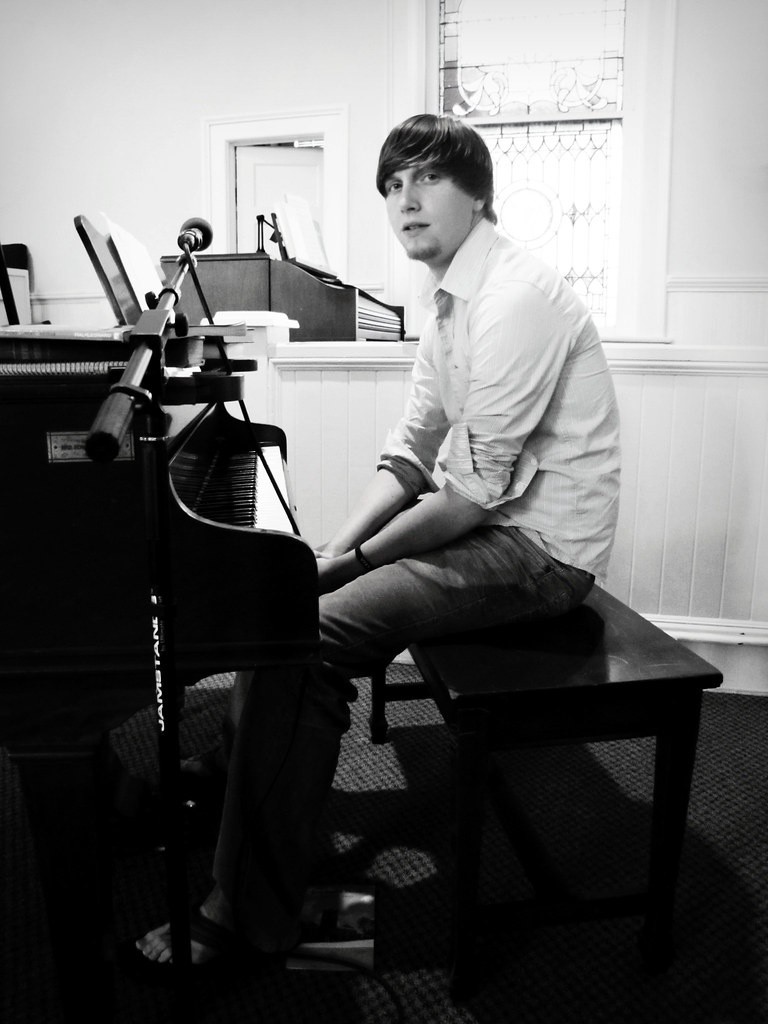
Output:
[157,254,407,344]
[0,211,323,1022]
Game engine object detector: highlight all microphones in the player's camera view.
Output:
[178,217,213,252]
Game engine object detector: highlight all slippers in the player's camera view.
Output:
[138,906,271,970]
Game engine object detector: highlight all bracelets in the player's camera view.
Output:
[355,542,375,572]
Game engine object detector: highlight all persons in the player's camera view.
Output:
[134,114,620,964]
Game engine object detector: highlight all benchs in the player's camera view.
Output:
[367,586,723,1003]
